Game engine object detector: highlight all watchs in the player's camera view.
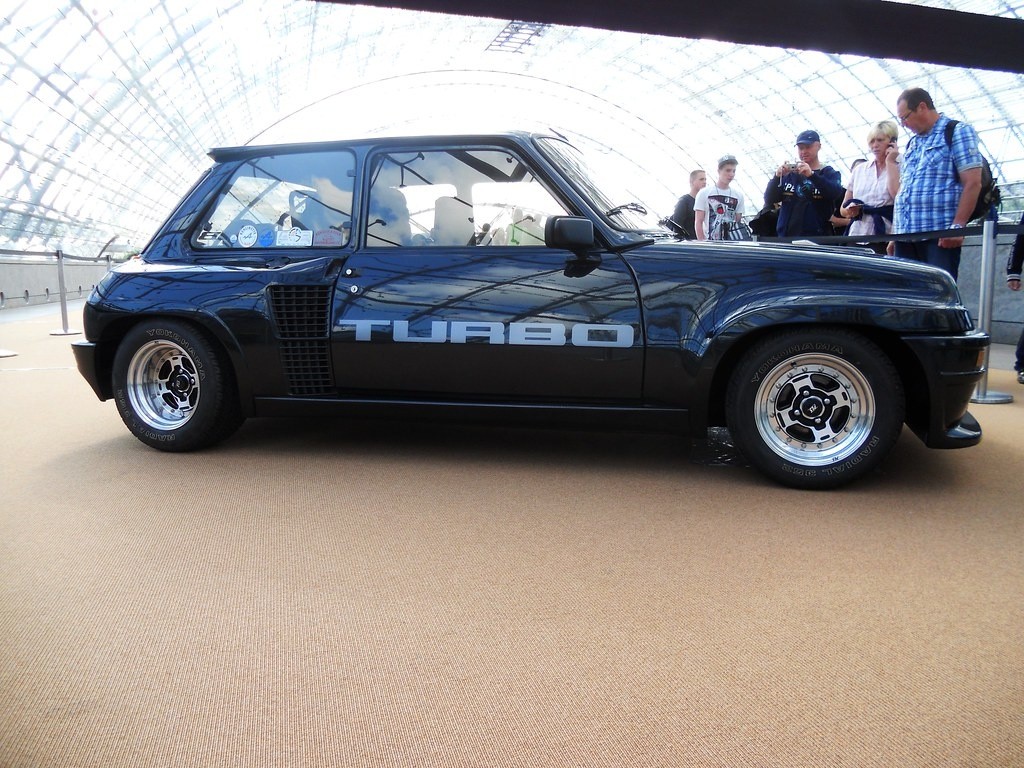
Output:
[949,223,963,232]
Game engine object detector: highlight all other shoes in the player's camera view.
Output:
[1017,372,1024,384]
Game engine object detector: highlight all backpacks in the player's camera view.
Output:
[906,119,1001,225]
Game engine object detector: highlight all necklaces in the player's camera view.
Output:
[714,182,732,213]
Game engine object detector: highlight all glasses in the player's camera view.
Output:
[901,106,918,121]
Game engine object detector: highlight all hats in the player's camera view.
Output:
[793,129,820,147]
[718,155,738,169]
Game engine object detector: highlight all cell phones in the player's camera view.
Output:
[887,137,897,148]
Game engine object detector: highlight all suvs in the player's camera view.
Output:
[70,129,991,491]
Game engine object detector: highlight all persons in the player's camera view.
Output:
[693,154,746,242]
[1006,211,1024,386]
[762,120,904,254]
[670,170,706,241]
[886,87,982,285]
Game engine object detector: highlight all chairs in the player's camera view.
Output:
[289,189,335,231]
[434,196,475,246]
[369,187,414,245]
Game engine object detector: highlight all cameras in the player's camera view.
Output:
[787,163,797,170]
